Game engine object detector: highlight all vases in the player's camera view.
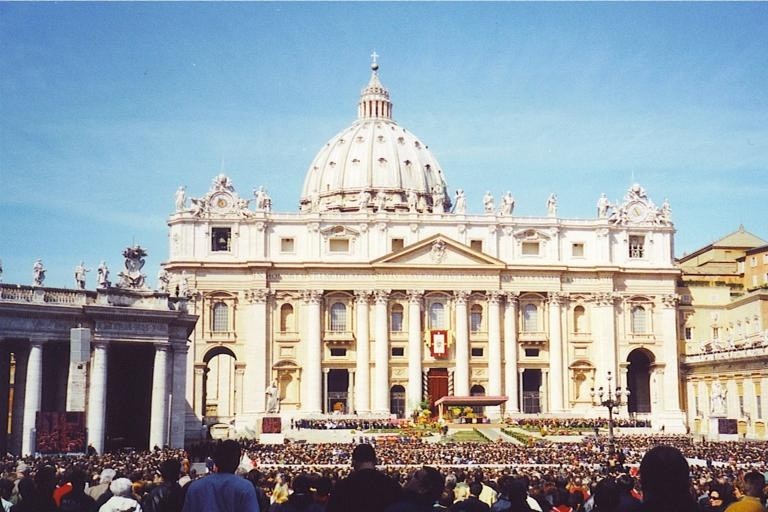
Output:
[465,418,472,423]
[477,418,482,423]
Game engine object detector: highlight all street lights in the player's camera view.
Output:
[589,370,634,458]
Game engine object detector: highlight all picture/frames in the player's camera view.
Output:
[431,330,448,358]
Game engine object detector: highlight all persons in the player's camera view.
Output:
[547,192,557,217]
[32,256,46,285]
[266,380,281,413]
[597,193,609,217]
[176,186,186,211]
[254,185,267,210]
[73,261,189,291]
[483,191,494,214]
[455,188,465,214]
[502,191,514,215]
[0,404,768,511]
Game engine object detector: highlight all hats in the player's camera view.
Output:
[16,464,33,474]
[349,444,374,468]
[155,458,182,482]
